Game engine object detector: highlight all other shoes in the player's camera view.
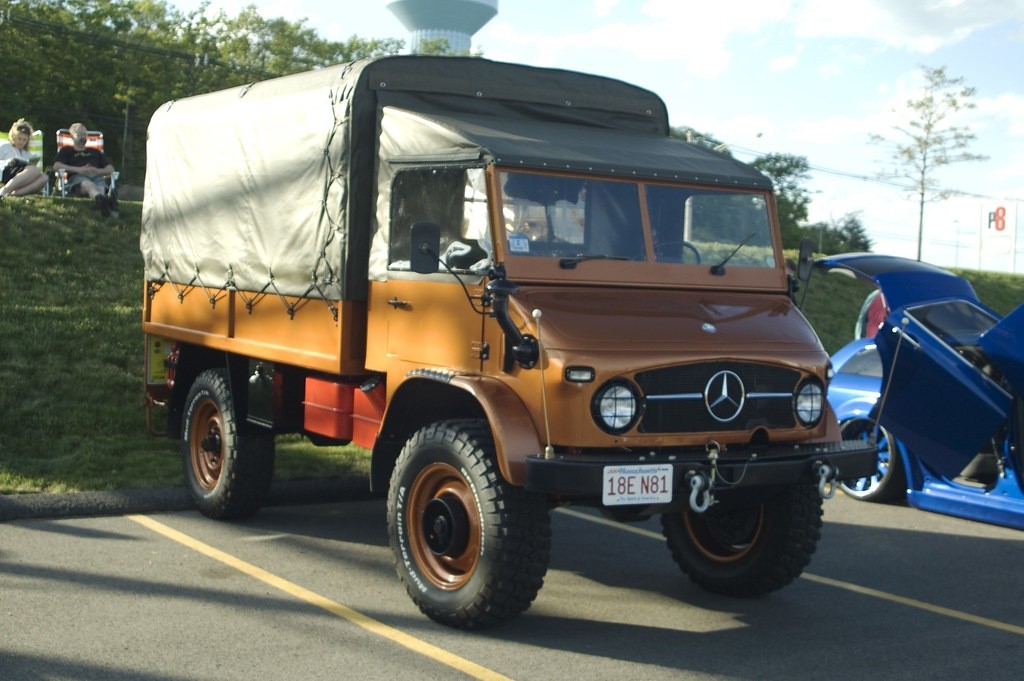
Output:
[107,188,120,211]
[94,193,111,217]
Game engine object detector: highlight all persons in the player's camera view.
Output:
[0,119,48,196]
[52,123,113,216]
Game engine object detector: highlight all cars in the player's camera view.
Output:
[813,252,1024,530]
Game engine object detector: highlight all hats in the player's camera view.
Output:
[17,125,30,134]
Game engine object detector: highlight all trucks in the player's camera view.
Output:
[141,55,881,634]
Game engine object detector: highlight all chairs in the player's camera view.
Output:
[47,129,119,200]
[0,130,53,197]
